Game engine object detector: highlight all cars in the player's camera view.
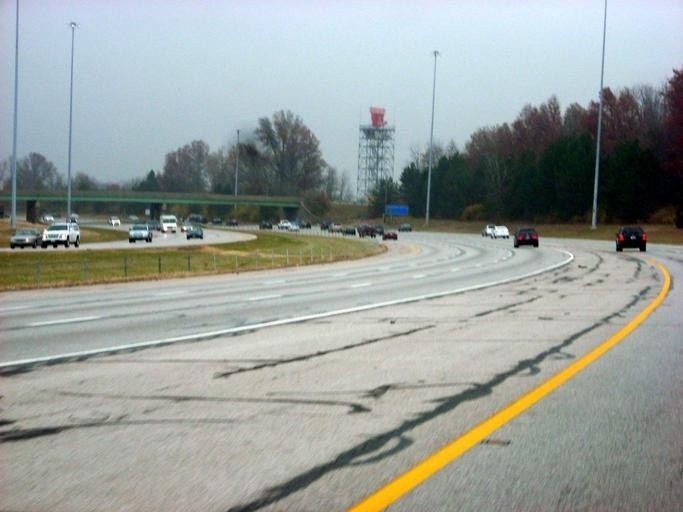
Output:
[108,215,121,227]
[128,213,238,243]
[482,224,509,240]
[43,215,56,224]
[513,228,539,248]
[616,226,647,252]
[66,213,80,223]
[398,223,412,232]
[259,219,398,241]
[10,223,81,249]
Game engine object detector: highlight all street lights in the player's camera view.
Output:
[424,50,439,224]
[67,20,77,216]
[234,129,240,196]
